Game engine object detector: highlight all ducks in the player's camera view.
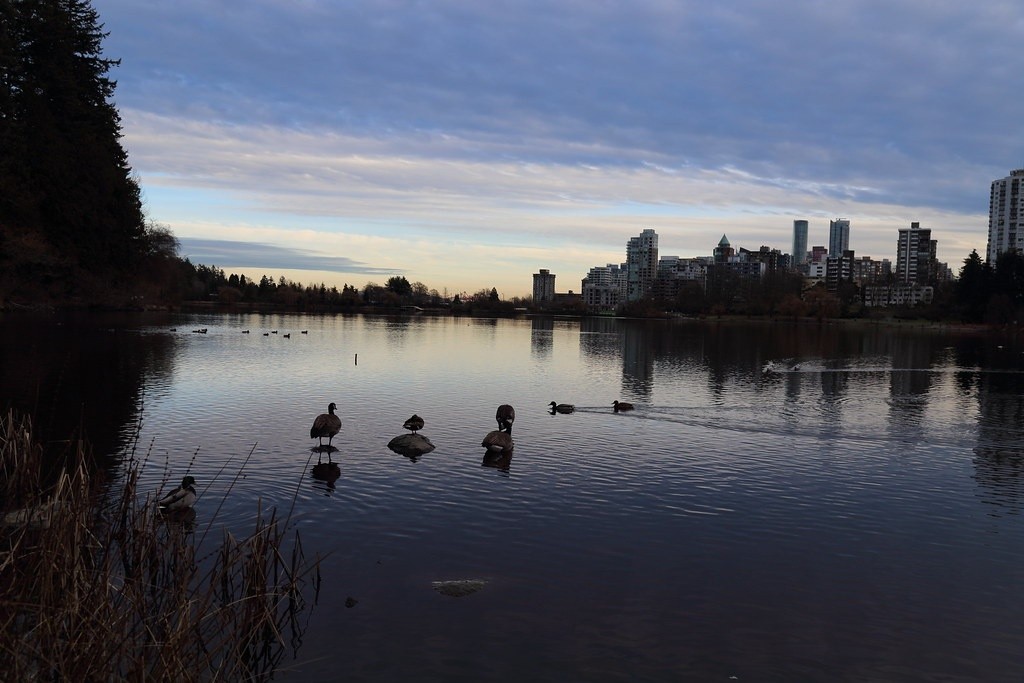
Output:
[481,420,514,453]
[310,402,342,446]
[151,476,202,509]
[611,399,633,410]
[402,414,424,434]
[548,401,575,414]
[496,404,515,432]
